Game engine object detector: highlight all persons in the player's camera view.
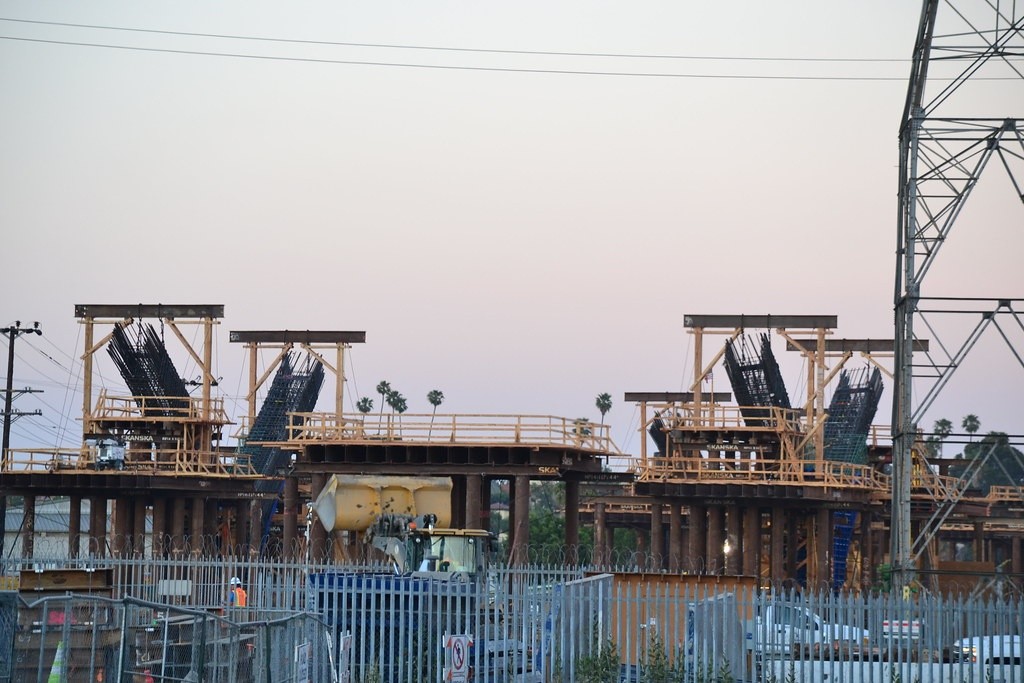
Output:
[229,577,248,609]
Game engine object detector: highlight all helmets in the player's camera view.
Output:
[231,577,242,585]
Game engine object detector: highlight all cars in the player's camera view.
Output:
[954,635,1022,667]
[733,601,869,655]
